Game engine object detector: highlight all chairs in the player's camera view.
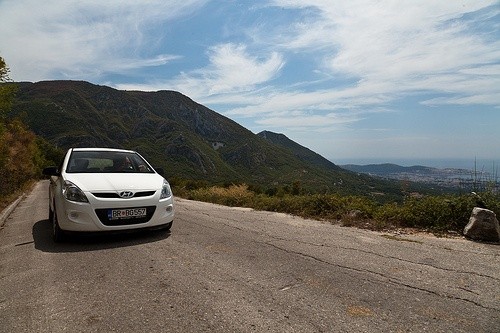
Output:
[70,158,91,172]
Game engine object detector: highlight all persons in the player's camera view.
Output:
[116,154,147,172]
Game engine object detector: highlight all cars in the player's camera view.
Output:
[42,147,174,241]
